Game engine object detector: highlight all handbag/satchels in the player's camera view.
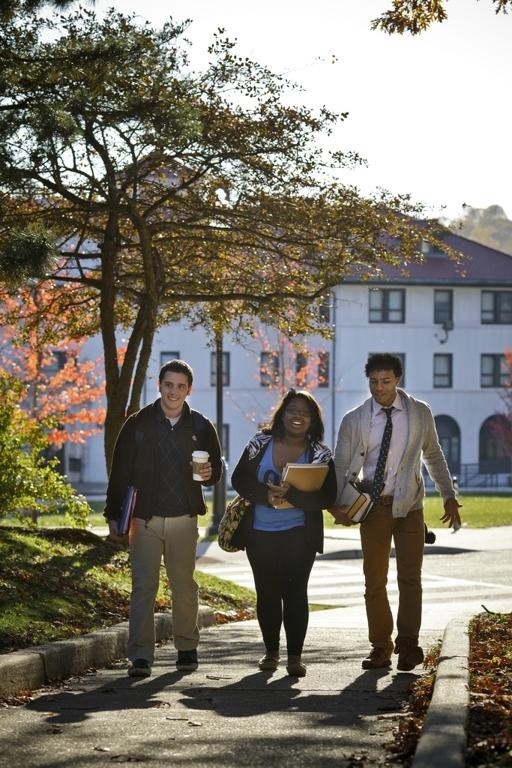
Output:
[218,495,252,552]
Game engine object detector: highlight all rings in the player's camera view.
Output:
[271,496,275,501]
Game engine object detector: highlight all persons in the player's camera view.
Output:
[102,357,223,678]
[229,386,337,679]
[328,352,465,674]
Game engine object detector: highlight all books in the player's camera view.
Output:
[333,476,375,527]
[270,462,331,510]
[116,486,138,537]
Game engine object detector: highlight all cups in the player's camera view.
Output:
[191,450,210,482]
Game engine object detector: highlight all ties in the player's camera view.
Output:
[370,406,395,504]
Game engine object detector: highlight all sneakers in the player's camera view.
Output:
[288,654,308,676]
[258,651,281,670]
[176,649,198,671]
[128,656,153,677]
[397,638,418,670]
[362,648,390,669]
